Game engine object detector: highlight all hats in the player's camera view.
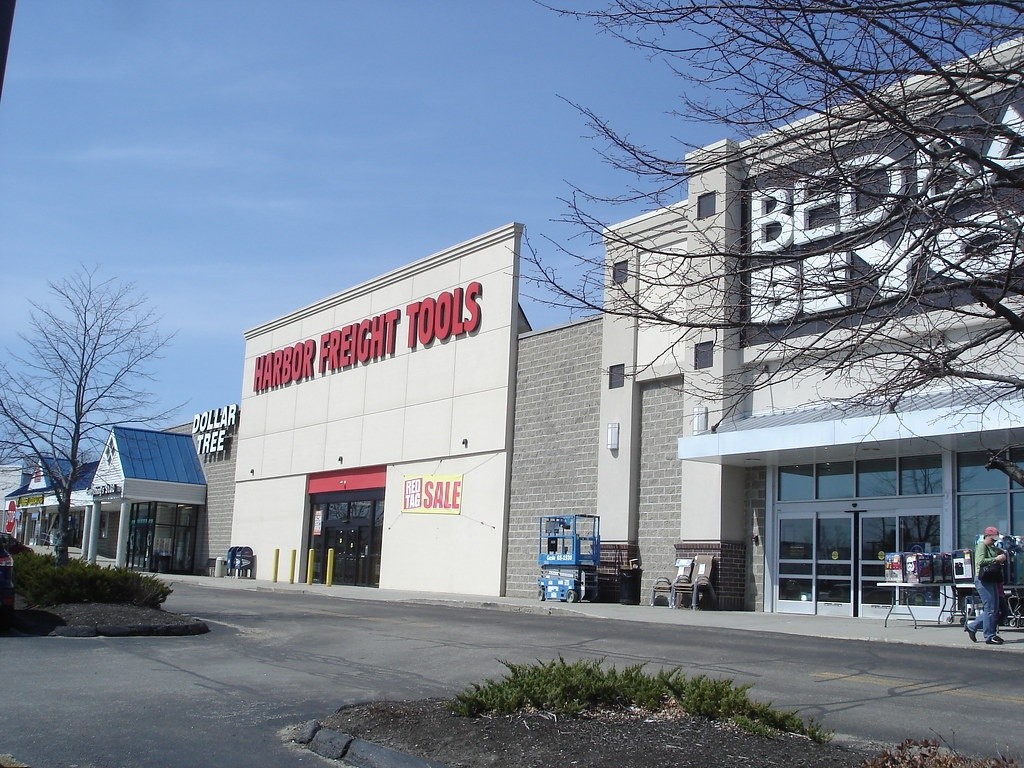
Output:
[984,526,1001,541]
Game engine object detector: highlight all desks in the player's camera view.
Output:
[877,581,1024,635]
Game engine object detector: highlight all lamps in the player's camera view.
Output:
[607,423,619,451]
[693,407,708,436]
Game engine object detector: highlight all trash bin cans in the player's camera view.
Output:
[227,546,253,577]
[214,556,228,578]
[157,555,172,573]
[618,568,642,606]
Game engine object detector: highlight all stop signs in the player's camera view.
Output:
[4,499,16,535]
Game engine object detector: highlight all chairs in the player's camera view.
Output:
[642,555,718,611]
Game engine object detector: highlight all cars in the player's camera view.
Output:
[0,535,16,634]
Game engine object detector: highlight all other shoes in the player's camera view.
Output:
[986,636,1004,644]
[965,626,977,642]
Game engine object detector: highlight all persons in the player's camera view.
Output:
[964,527,1007,645]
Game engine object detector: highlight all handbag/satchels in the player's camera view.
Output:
[978,563,1004,581]
